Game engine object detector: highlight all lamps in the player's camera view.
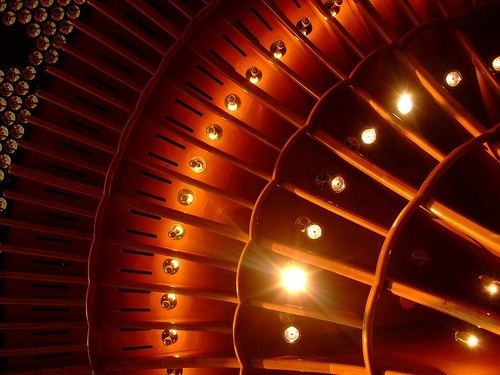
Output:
[1,2,500,347]
